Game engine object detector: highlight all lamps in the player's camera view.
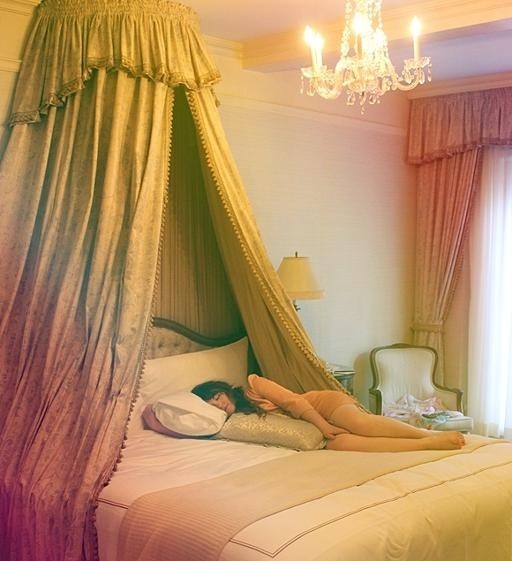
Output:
[299,0,433,115]
[275,252,323,314]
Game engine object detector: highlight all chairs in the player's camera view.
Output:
[369,343,463,423]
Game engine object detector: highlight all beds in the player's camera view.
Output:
[1,314,512,561]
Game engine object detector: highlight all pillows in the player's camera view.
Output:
[137,336,250,407]
[142,402,183,438]
[152,389,227,435]
[212,413,327,453]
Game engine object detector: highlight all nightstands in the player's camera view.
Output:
[330,371,356,398]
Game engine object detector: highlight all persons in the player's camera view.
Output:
[191,374,466,453]
